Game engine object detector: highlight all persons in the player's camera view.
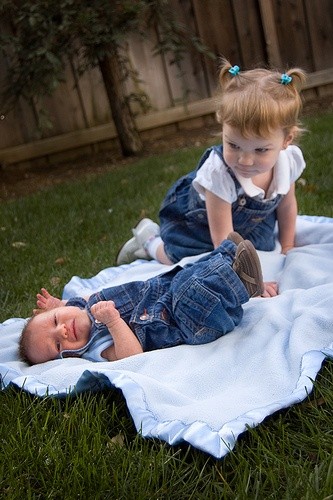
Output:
[18,232,264,366]
[117,53,309,297]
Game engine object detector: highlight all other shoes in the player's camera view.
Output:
[117,217,162,263]
[225,230,265,298]
